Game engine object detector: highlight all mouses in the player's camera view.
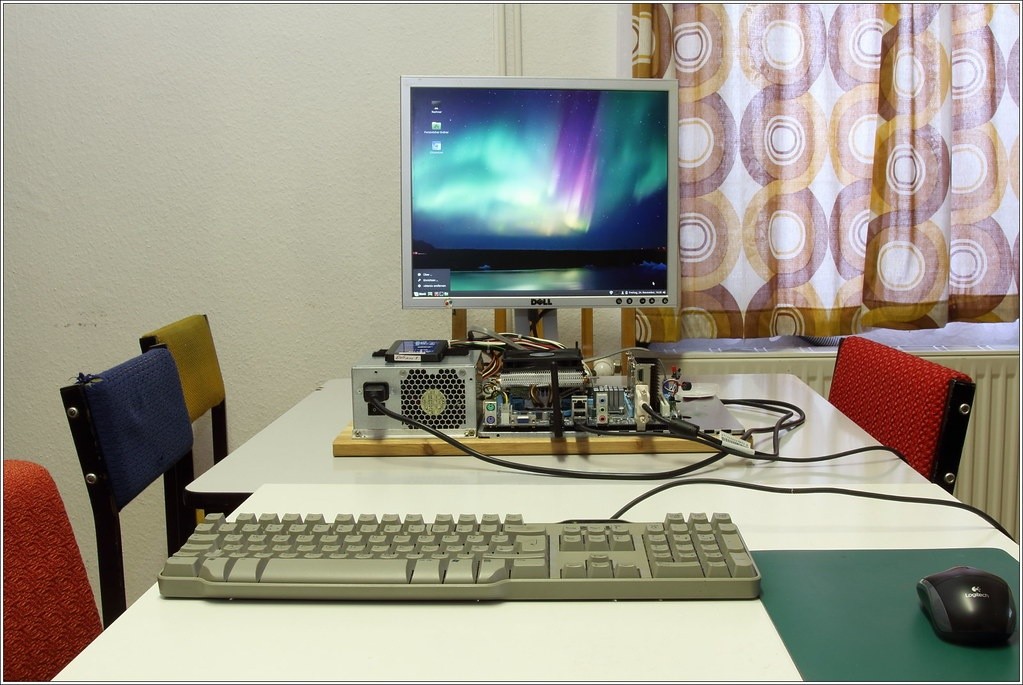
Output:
[917,564,1018,645]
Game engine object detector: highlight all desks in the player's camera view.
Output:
[50,482,1020,682]
[183,374,929,507]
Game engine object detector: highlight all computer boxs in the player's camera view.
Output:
[351,352,747,440]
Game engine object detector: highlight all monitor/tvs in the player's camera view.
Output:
[398,76,679,310]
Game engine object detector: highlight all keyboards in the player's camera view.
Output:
[157,511,763,602]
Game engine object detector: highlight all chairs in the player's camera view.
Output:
[3,459,103,682]
[60,342,194,632]
[138,313,227,533]
[828,336,974,496]
